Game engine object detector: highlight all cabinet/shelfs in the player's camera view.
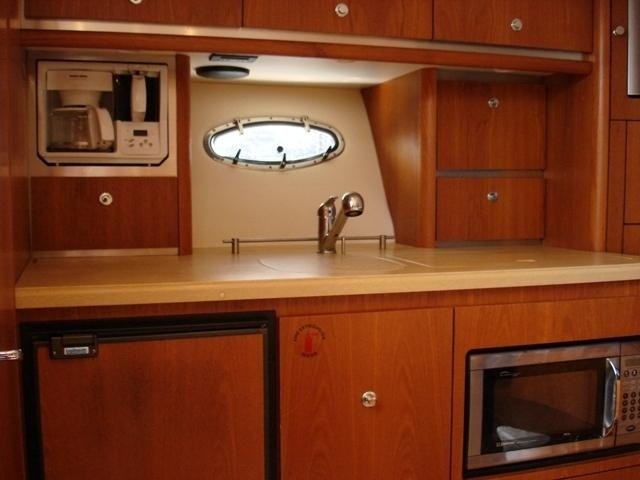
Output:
[361,69,550,246]
[277,290,454,475]
[242,0,432,49]
[12,298,279,480]
[432,2,595,60]
[16,1,242,38]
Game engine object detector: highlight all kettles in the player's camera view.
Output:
[47,106,115,152]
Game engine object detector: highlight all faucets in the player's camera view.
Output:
[318,192,364,255]
